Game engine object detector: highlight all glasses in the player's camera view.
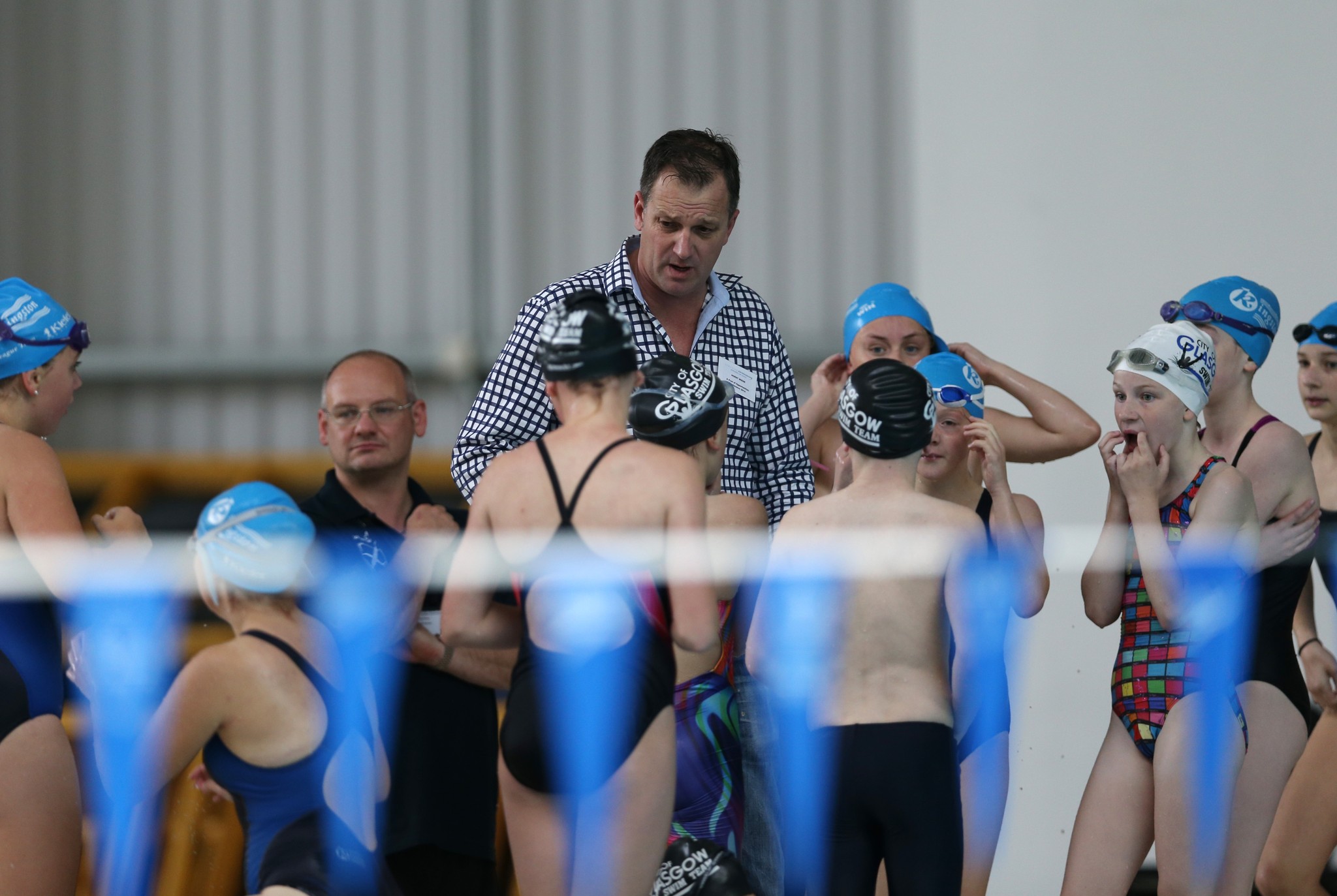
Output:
[1106,348,1158,373]
[932,385,973,409]
[721,380,735,407]
[69,322,91,351]
[322,400,413,425]
[1293,324,1337,348]
[1160,301,1215,324]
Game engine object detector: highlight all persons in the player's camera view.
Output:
[830,352,1050,896]
[1159,276,1322,896]
[449,127,814,896]
[744,359,999,896]
[1257,302,1337,896]
[796,282,1101,498]
[1059,320,1259,896]
[0,275,154,896]
[440,289,720,896]
[295,349,522,896]
[64,481,389,896]
[626,353,771,896]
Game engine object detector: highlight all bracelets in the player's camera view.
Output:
[439,644,454,670]
[1298,638,1322,657]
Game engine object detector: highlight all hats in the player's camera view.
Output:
[911,351,985,418]
[195,482,315,596]
[535,290,634,372]
[843,282,949,363]
[1114,320,1216,418]
[627,351,728,450]
[1298,301,1337,351]
[0,277,74,379]
[1175,276,1281,367]
[837,358,936,460]
